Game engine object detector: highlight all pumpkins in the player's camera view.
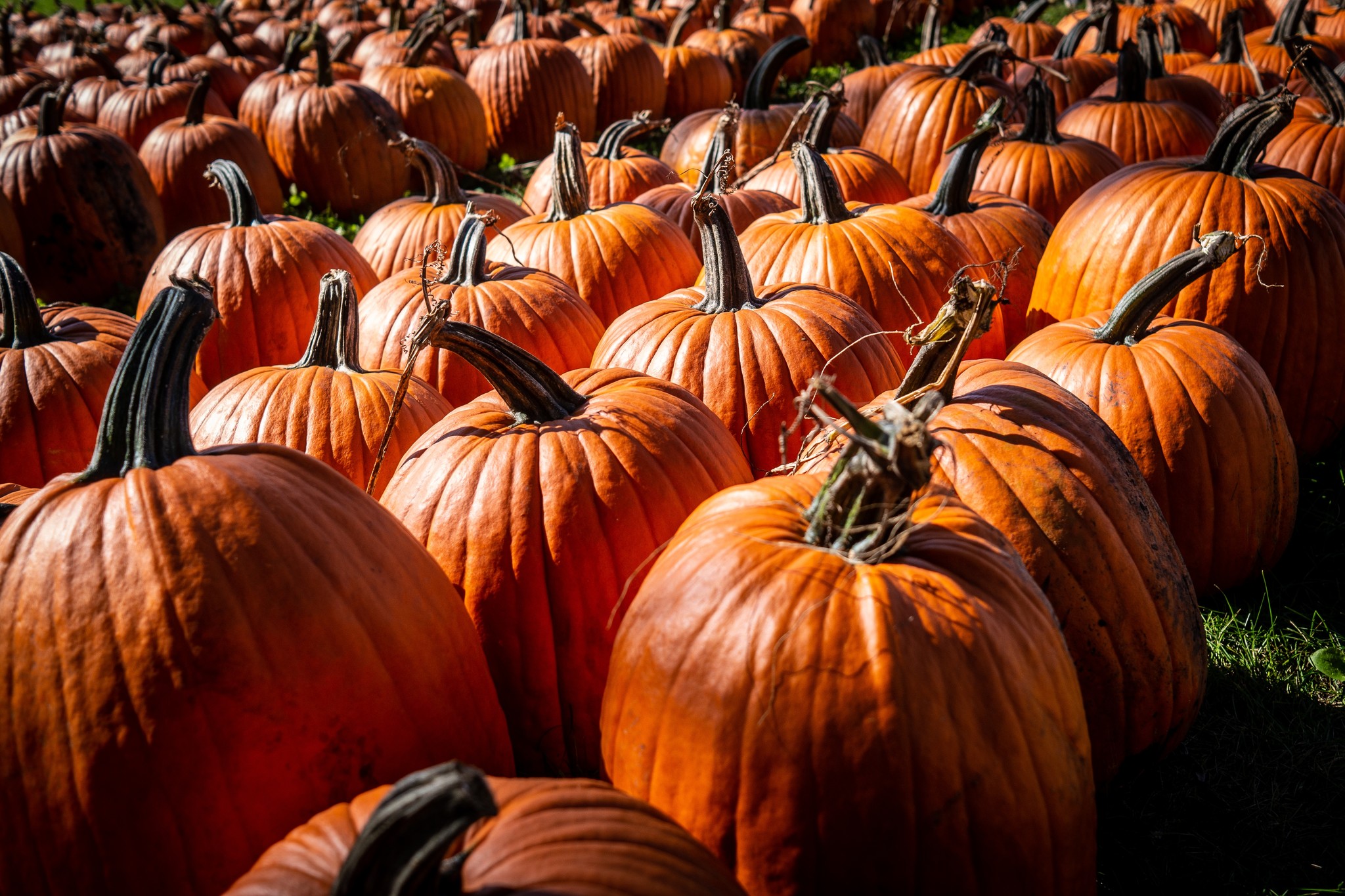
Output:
[1,0,1343,896]
